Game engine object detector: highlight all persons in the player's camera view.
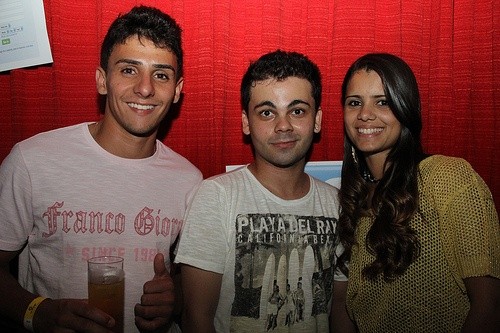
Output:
[1,6,203,333]
[336,52,500,333]
[170,49,344,333]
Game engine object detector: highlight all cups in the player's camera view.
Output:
[87,256,124,333]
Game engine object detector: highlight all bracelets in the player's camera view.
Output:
[23,295,49,333]
